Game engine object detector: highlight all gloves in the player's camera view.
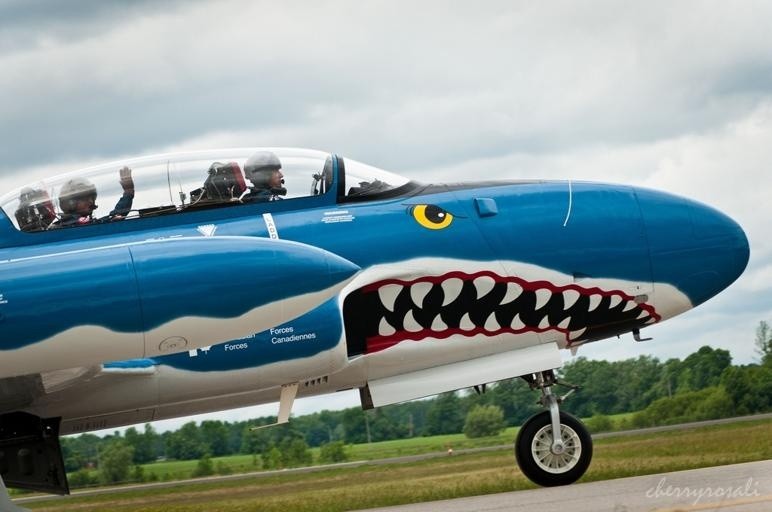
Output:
[118,166,135,199]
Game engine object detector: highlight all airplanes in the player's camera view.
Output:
[1,138,750,497]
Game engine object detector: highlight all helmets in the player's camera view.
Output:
[244,150,282,180]
[57,176,97,201]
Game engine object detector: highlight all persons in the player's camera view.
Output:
[48,167,134,230]
[241,150,287,204]
